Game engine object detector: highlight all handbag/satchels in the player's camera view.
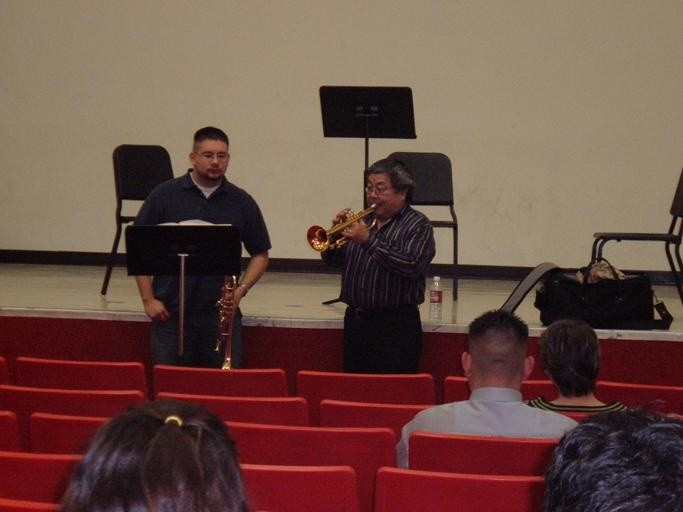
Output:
[536,258,673,329]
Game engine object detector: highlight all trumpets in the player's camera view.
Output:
[309,204,379,252]
[213,275,236,369]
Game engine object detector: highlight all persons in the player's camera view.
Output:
[392,307,584,473]
[317,155,437,373]
[44,397,247,511]
[533,403,682,510]
[522,319,632,423]
[124,122,272,369]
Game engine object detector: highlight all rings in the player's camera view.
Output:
[348,229,351,233]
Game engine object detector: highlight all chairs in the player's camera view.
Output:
[387,152,459,303]
[590,167,683,306]
[100,144,174,296]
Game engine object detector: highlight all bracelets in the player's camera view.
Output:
[235,282,248,298]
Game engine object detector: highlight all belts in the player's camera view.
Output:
[349,306,401,322]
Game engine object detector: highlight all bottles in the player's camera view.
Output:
[429,274,443,325]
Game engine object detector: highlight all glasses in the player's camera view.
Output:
[364,185,397,194]
[193,151,230,161]
